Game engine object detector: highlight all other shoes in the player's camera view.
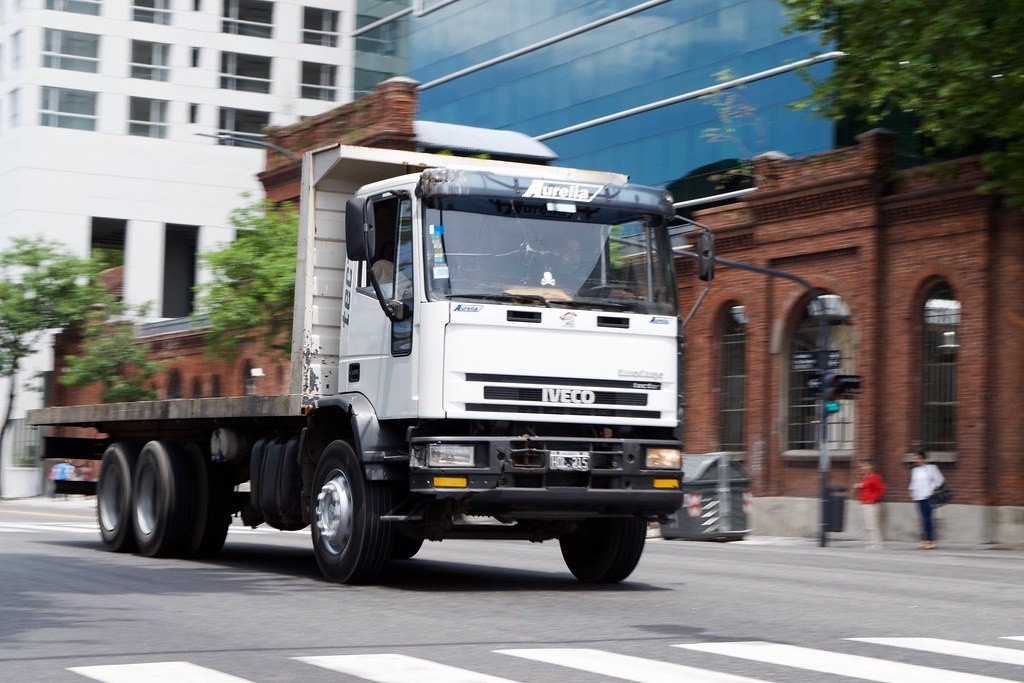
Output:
[865,545,881,550]
[914,542,938,550]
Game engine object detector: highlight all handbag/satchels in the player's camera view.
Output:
[929,487,947,509]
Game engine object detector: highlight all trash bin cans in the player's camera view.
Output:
[659,452,754,542]
[826,485,849,533]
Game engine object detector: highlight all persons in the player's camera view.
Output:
[907,451,944,549]
[535,235,634,299]
[855,459,888,550]
[48,459,93,501]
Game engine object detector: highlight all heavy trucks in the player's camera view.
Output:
[24,142,718,585]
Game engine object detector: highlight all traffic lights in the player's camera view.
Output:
[825,373,844,414]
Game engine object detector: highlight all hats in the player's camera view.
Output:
[857,464,872,470]
